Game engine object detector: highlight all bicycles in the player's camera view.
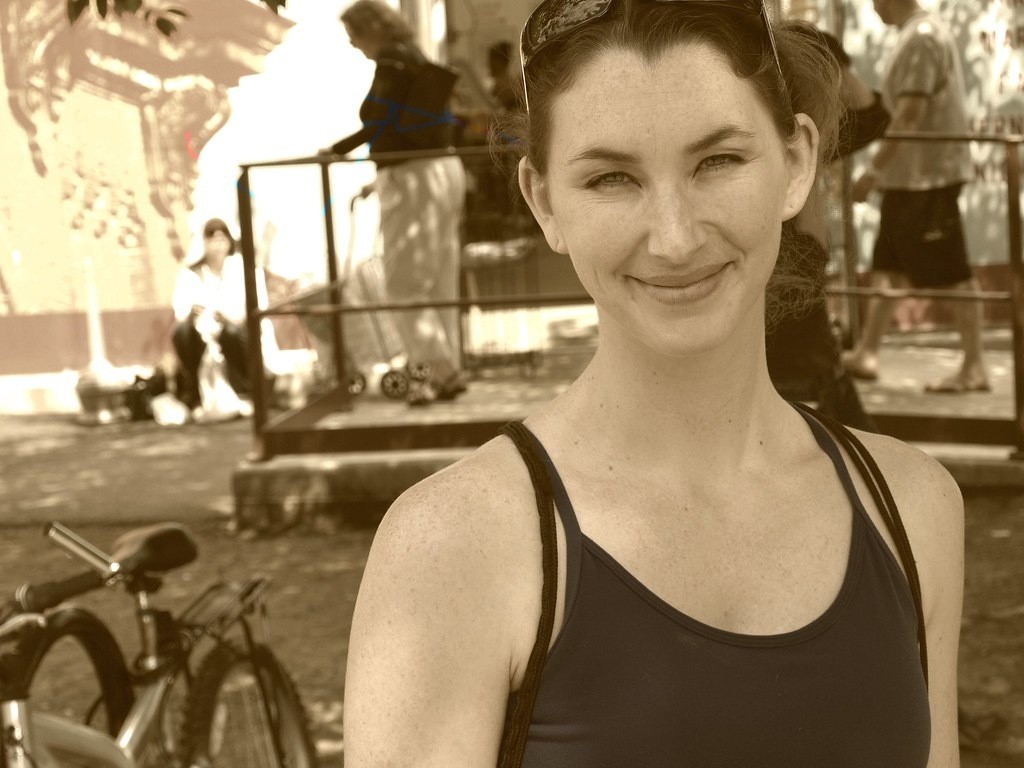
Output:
[0,522,320,768]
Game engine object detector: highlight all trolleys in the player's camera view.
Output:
[291,185,410,400]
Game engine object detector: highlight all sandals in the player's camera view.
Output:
[408,369,469,405]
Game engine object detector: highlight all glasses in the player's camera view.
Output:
[520,0,792,155]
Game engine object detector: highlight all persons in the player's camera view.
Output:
[831,0,993,392]
[763,24,895,433]
[170,213,289,415]
[319,0,470,403]
[342,0,963,768]
